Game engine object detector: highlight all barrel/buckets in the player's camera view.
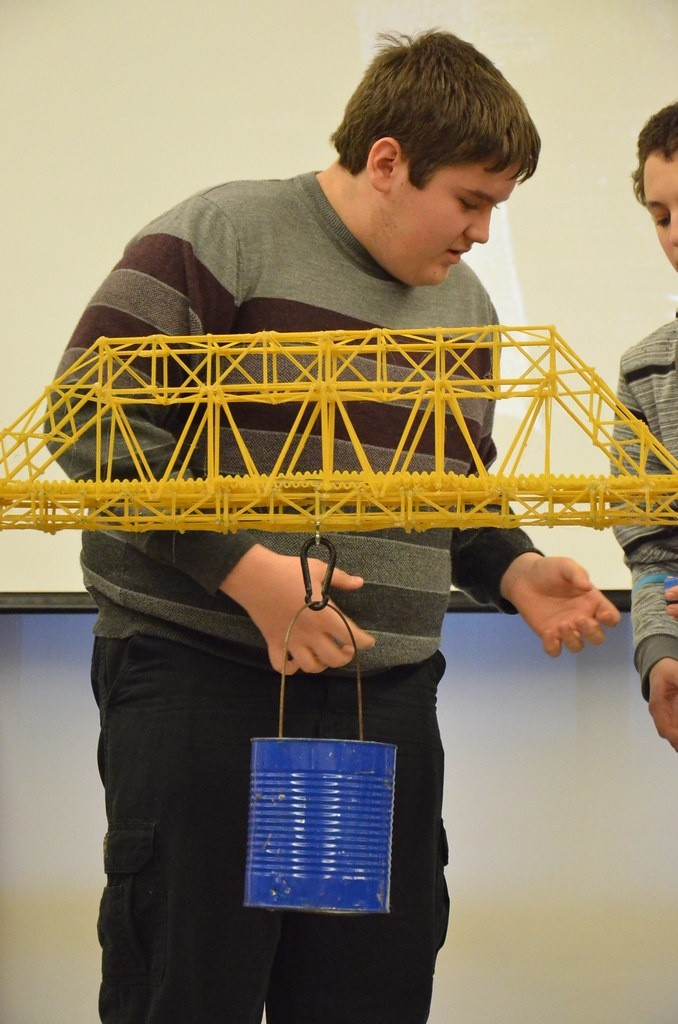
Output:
[239,736,399,912]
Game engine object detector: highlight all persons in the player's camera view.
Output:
[611,104,678,749]
[42,24,622,1023]
